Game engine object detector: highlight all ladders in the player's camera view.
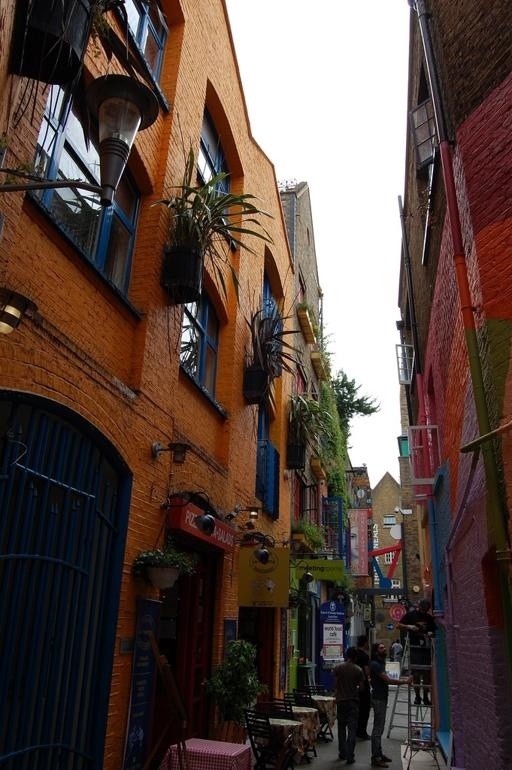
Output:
[387,632,436,739]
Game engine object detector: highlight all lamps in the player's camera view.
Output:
[149,441,192,464]
[289,560,314,584]
[1,287,40,335]
[160,490,216,537]
[248,531,280,573]
[1,72,159,206]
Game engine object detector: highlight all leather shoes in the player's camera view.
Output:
[372,756,392,768]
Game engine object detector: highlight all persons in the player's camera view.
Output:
[369,642,413,768]
[389,642,395,662]
[396,600,438,704]
[332,646,366,764]
[351,634,372,740]
[391,639,403,662]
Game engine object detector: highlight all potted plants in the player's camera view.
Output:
[149,112,272,306]
[287,393,334,470]
[243,309,309,405]
[132,545,199,590]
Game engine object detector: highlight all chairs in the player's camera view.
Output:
[244,685,335,769]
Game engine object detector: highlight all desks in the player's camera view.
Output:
[159,738,251,770]
